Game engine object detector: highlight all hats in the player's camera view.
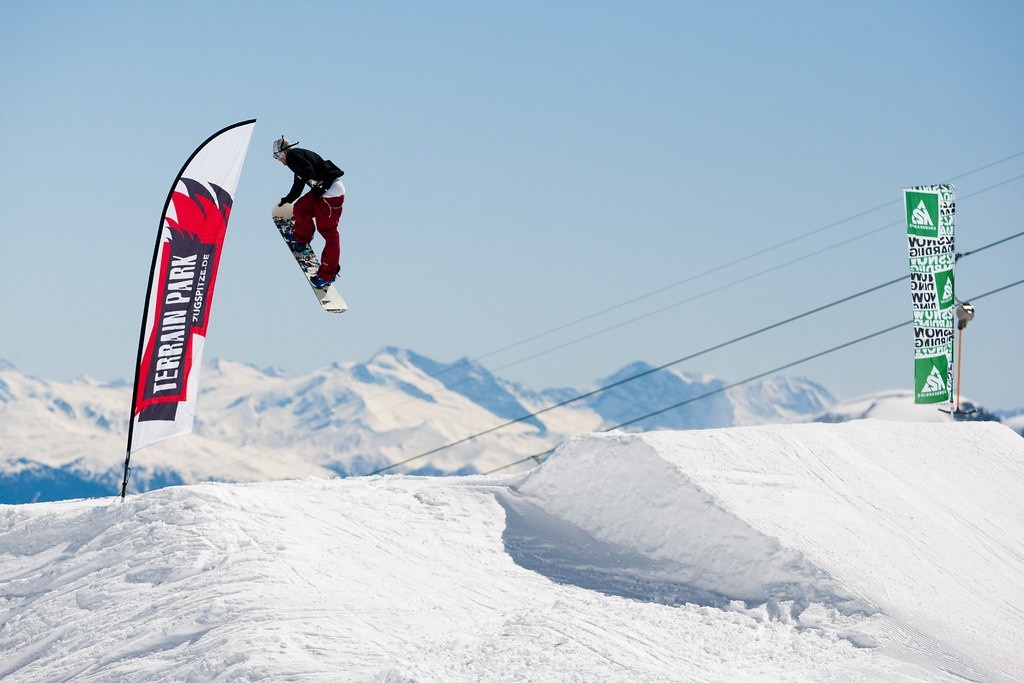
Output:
[274,138,294,157]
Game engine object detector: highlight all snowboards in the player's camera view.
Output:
[271,201,348,315]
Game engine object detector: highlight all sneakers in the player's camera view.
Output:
[284,231,297,243]
[309,274,330,288]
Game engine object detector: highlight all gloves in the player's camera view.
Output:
[278,197,289,207]
[314,184,326,196]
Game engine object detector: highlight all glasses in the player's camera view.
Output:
[274,152,282,159]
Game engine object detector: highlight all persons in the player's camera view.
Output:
[271,134,346,291]
